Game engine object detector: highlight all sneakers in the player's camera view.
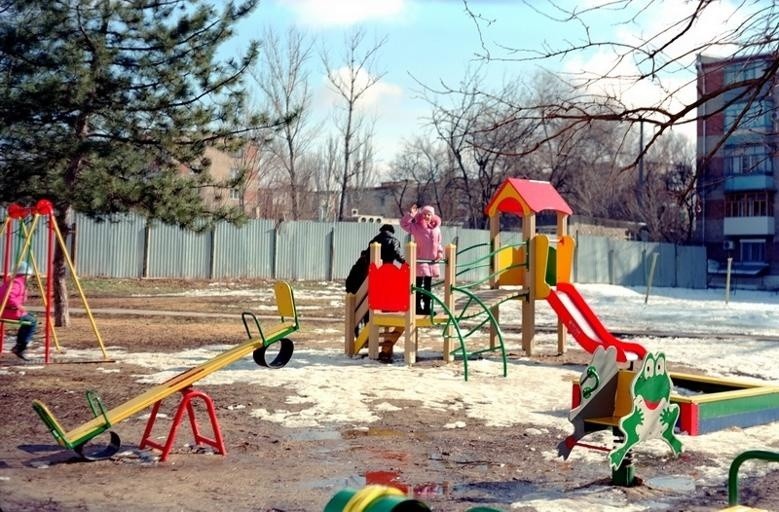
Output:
[11,342,31,362]
[416,303,436,315]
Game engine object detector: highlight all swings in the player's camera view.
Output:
[0,209,38,324]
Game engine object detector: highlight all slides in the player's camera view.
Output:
[545,283,646,362]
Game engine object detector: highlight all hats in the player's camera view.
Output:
[379,224,395,235]
[421,205,435,214]
[15,261,34,276]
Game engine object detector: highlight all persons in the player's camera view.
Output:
[399,203,444,317]
[0,260,38,362]
[345,224,409,341]
